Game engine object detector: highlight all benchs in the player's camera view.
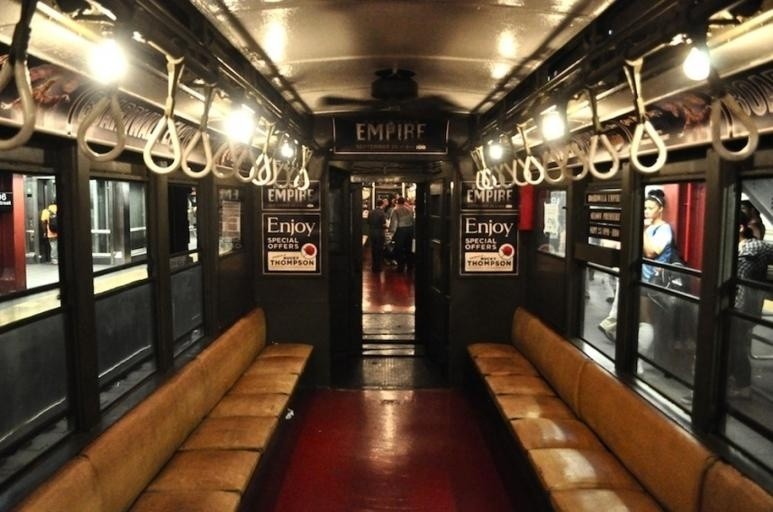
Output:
[464,306,772,511]
[2,306,313,512]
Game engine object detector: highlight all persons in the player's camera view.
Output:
[40,199,59,266]
[586,189,673,376]
[367,192,415,276]
[724,200,773,402]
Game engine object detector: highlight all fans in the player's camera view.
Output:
[312,66,473,158]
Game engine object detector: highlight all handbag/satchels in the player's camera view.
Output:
[648,262,689,312]
